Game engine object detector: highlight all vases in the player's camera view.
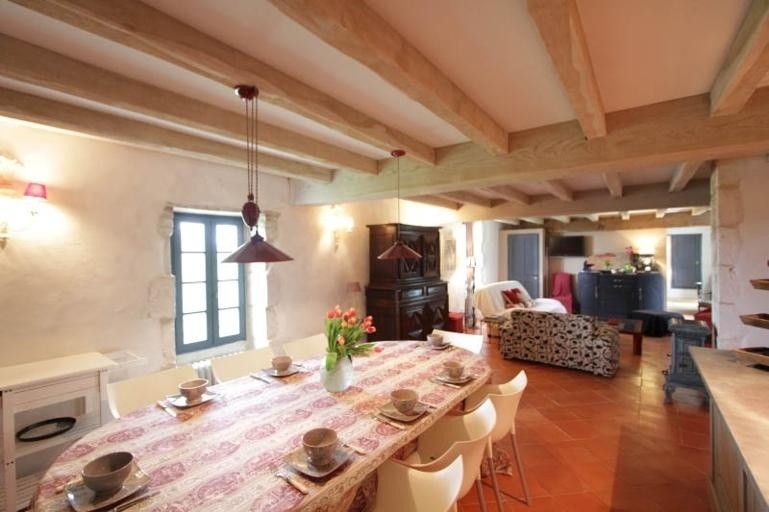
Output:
[320,355,354,393]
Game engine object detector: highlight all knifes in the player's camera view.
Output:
[155,399,178,419]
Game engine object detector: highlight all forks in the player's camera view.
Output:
[266,461,311,496]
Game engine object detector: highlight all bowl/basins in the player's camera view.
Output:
[301,426,341,465]
[79,450,137,498]
[177,377,210,403]
[270,354,294,372]
[389,386,421,414]
[426,333,444,346]
[441,360,466,380]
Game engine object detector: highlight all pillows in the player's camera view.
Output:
[501,289,521,307]
[512,287,536,307]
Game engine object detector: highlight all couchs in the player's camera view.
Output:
[549,272,573,314]
[499,308,623,378]
[473,280,566,318]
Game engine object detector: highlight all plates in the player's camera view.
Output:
[428,373,479,391]
[374,402,432,425]
[260,366,301,380]
[163,392,223,409]
[14,416,78,443]
[277,446,358,482]
[61,473,155,512]
[418,340,453,352]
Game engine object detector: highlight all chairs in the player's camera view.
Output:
[390,397,498,512]
[374,453,468,511]
[458,370,532,512]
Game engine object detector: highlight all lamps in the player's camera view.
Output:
[222,84,293,264]
[376,150,422,260]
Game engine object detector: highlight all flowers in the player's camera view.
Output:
[324,305,383,373]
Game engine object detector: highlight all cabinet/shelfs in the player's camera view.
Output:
[577,271,665,322]
[364,223,448,339]
[499,229,549,301]
[0,350,117,512]
[665,225,713,313]
[688,347,768,511]
